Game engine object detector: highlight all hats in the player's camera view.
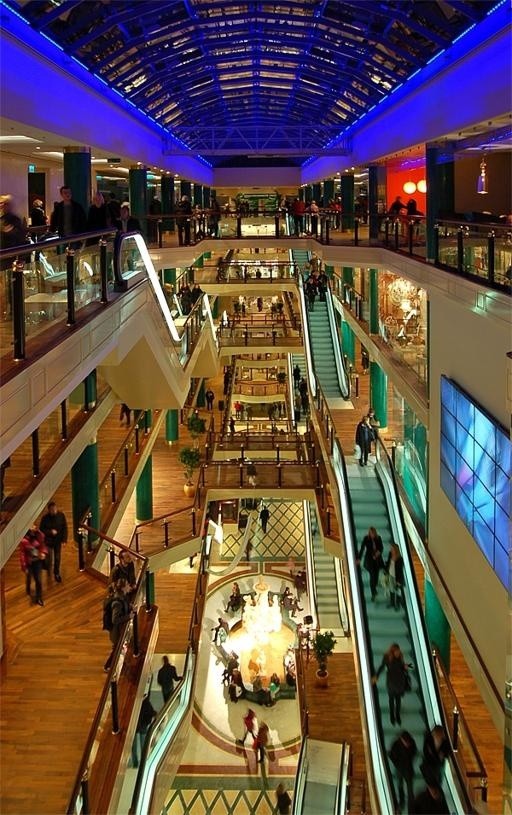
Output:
[428,778,441,790]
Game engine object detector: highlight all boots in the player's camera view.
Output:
[358,456,364,467]
[393,593,401,612]
[363,456,368,465]
[387,592,395,608]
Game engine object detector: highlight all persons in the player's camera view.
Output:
[109,549,136,595]
[256,268,262,280]
[42,502,68,582]
[303,257,328,312]
[158,655,185,705]
[367,407,380,453]
[223,364,312,434]
[284,196,421,236]
[356,415,372,467]
[212,568,311,762]
[120,404,132,427]
[104,578,136,675]
[233,296,282,317]
[137,691,160,755]
[260,506,270,534]
[20,525,49,606]
[0,185,222,320]
[247,461,256,487]
[354,527,455,815]
[206,387,216,412]
[236,271,241,280]
[246,539,252,561]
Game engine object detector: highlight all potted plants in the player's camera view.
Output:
[312,630,337,686]
[178,446,201,499]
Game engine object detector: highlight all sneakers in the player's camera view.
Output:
[103,665,112,673]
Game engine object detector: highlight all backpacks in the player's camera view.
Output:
[102,597,125,631]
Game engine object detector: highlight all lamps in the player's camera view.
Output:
[383,275,418,316]
[402,181,417,195]
[244,575,284,666]
[477,154,489,196]
[416,178,427,194]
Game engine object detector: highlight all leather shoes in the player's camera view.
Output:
[395,710,401,727]
[36,597,43,606]
[390,711,395,727]
[54,572,62,582]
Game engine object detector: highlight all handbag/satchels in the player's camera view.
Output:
[41,556,50,571]
[369,429,375,441]
[403,666,413,694]
[381,572,389,587]
[370,419,381,427]
[352,444,361,460]
